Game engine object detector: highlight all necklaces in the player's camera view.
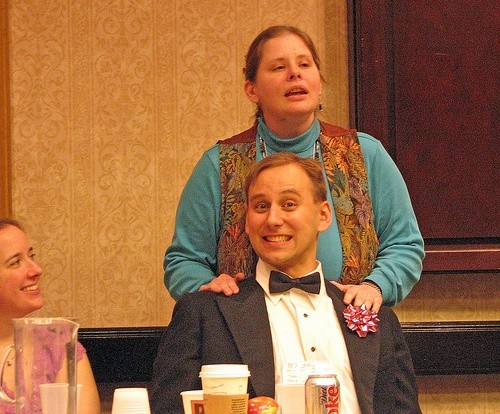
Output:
[259,135,321,161]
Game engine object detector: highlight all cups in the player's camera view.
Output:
[112,388,150,414]
[199,364,251,414]
[179,390,205,414]
[12,317,82,414]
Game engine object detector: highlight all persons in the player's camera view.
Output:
[148,152,422,414]
[163,24,426,317]
[0,219,101,414]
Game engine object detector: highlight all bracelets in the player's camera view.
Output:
[359,281,382,294]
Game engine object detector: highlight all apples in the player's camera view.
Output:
[247,396,279,414]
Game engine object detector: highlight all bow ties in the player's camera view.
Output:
[269,270,321,294]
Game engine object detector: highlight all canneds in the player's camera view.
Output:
[305,373,341,414]
